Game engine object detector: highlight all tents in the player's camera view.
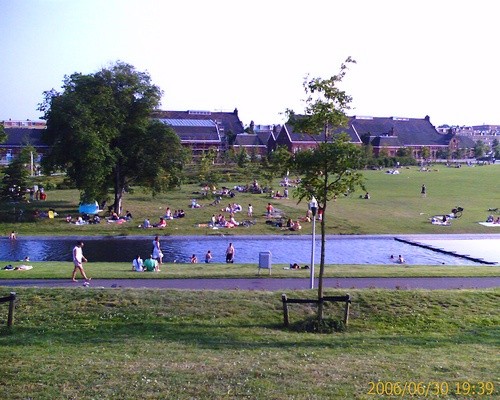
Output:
[79,198,99,214]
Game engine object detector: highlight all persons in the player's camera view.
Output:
[66,212,100,225]
[486,214,500,225]
[2,256,30,271]
[9,230,16,239]
[131,235,309,284]
[137,172,350,233]
[431,207,457,225]
[71,241,91,282]
[390,253,406,263]
[101,200,132,223]
[364,192,370,199]
[421,184,426,197]
[369,156,496,174]
[8,185,58,218]
